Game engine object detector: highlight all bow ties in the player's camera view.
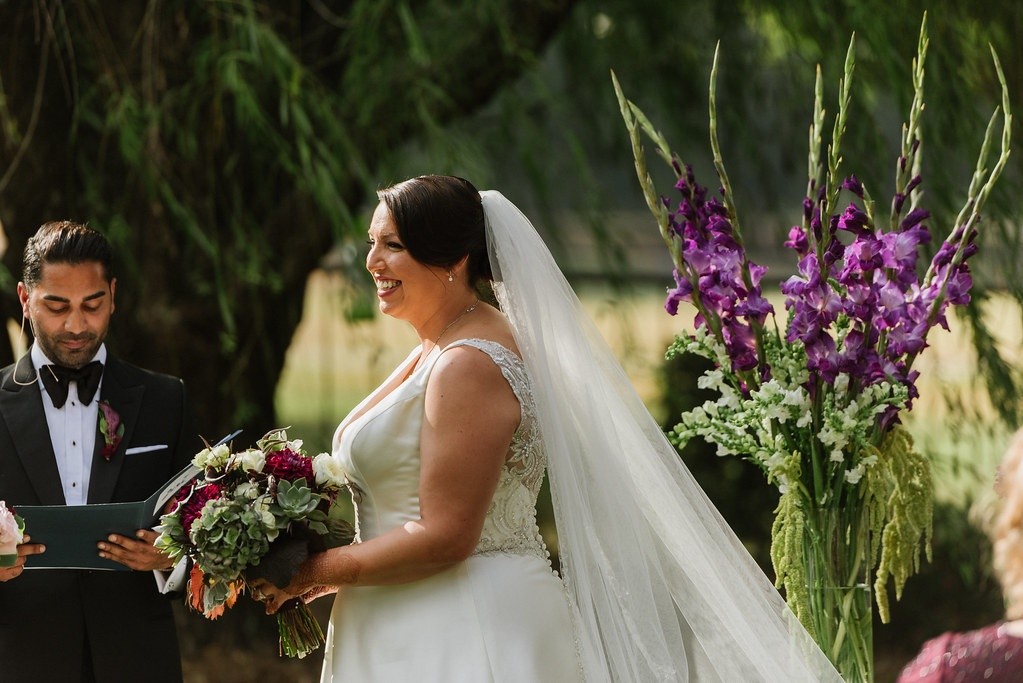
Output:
[39,360,105,410]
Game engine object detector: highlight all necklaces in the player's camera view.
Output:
[409,295,480,379]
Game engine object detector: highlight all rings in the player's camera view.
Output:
[257,587,267,599]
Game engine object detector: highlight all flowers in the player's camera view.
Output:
[0,500,25,567]
[152,426,354,660]
[611,7,1014,682]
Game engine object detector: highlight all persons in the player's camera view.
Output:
[0,220,193,683]
[247,174,585,683]
